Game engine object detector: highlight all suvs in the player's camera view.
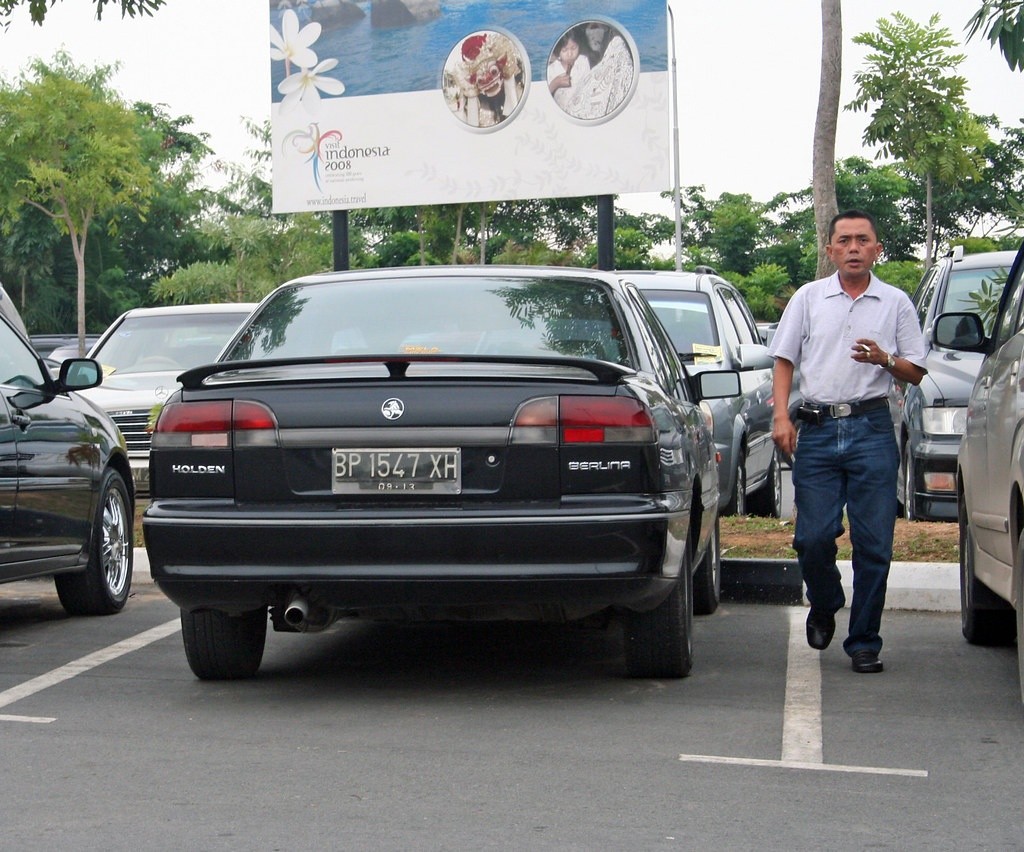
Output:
[602,264,785,519]
[896,244,1017,521]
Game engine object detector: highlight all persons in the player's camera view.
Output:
[546,22,634,121]
[767,210,928,674]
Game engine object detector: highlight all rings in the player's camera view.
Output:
[866,352,871,358]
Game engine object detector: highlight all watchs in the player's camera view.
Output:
[885,353,896,370]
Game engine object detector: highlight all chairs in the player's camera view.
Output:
[668,321,711,354]
[551,340,605,359]
[946,290,981,314]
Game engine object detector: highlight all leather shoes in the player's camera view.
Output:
[851,649,884,672]
[806,607,836,650]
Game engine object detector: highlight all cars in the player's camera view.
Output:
[47,303,258,498]
[929,244,1023,697]
[140,264,772,681]
[1,287,136,615]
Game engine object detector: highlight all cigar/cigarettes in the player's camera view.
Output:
[859,343,871,352]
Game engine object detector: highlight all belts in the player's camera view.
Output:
[797,397,890,418]
